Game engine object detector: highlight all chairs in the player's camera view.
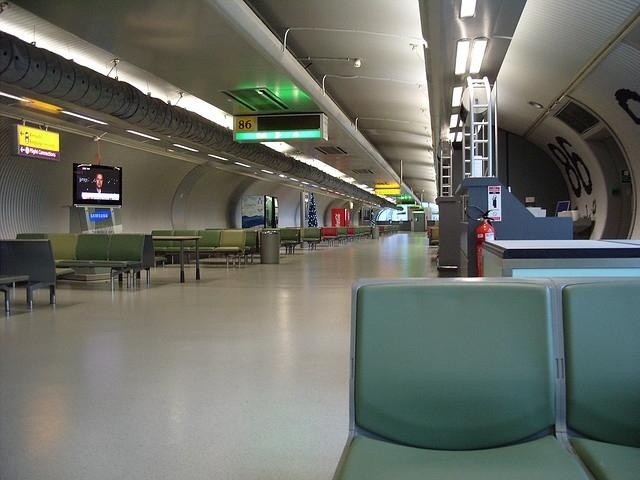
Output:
[148,229,260,267]
[16,230,152,294]
[277,225,402,254]
[1,238,60,313]
[334,277,639,480]
[427,226,439,253]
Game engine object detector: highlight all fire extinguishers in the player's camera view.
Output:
[474,210,496,276]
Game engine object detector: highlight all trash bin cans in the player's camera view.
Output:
[372,227,380,239]
[260,231,280,264]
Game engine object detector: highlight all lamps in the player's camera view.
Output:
[353,57,361,68]
[374,182,401,194]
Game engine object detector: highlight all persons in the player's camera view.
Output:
[88,172,110,193]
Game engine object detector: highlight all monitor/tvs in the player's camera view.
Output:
[72,163,123,208]
[555,201,571,213]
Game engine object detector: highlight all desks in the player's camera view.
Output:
[153,233,202,284]
